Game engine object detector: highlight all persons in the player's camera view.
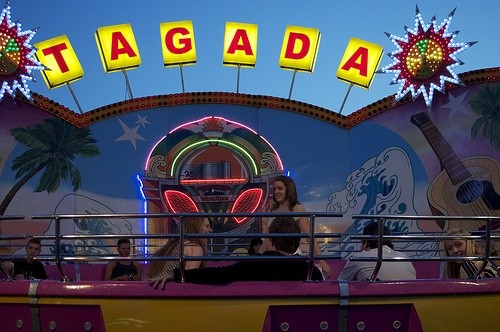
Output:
[145,213,325,292]
[103,237,141,281]
[336,220,418,282]
[443,226,500,279]
[3,238,49,282]
[257,174,333,278]
[247,238,264,254]
[146,211,215,279]
